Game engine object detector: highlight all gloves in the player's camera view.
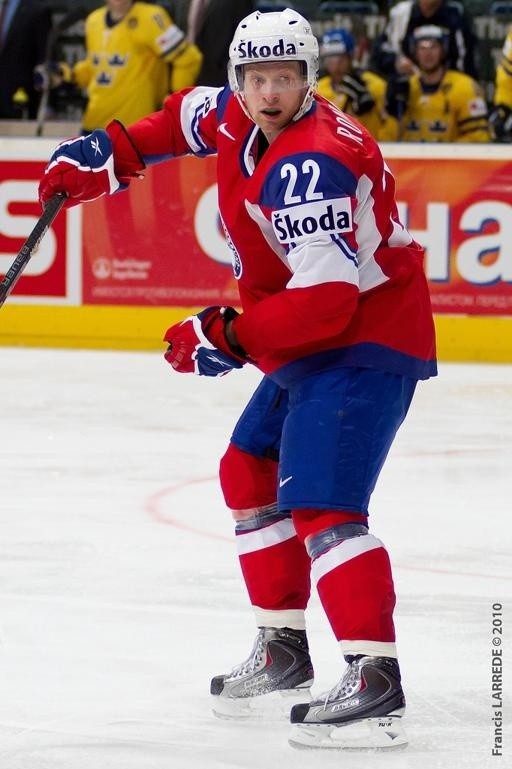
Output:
[164,305,250,379]
[339,73,374,117]
[384,75,410,116]
[38,119,146,209]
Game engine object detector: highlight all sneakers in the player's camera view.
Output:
[211,625,314,698]
[291,653,406,724]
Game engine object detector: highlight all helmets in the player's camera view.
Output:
[409,25,452,61]
[227,8,320,124]
[321,28,355,67]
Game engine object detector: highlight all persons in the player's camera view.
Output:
[39,3,439,723]
[316,0,512,145]
[33,0,204,134]
